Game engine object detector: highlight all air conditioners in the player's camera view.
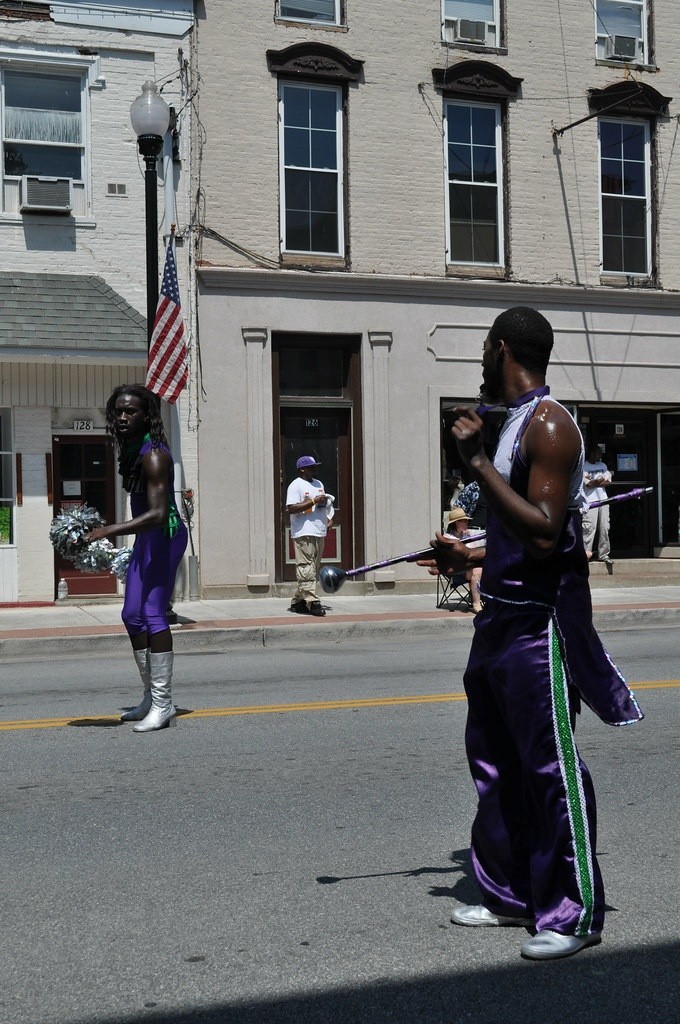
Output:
[453,18,489,44]
[604,34,638,62]
[17,174,73,216]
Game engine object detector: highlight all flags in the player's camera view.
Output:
[145,238,189,405]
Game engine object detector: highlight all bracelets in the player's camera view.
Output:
[312,498,315,504]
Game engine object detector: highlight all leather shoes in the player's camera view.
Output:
[602,556,614,565]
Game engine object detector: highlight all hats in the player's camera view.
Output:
[445,507,473,525]
[296,455,322,470]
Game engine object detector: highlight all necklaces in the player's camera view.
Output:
[476,386,550,416]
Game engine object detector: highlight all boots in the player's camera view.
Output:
[120,648,151,723]
[133,651,176,733]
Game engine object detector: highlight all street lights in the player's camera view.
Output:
[129,79,171,419]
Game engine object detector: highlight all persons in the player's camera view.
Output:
[583,443,614,564]
[453,479,487,530]
[451,305,641,958]
[286,456,335,616]
[442,507,482,613]
[43,384,187,731]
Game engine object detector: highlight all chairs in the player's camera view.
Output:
[436,573,474,612]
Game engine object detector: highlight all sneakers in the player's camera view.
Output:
[309,601,326,617]
[290,599,311,614]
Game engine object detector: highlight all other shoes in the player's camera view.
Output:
[520,927,605,959]
[451,904,538,928]
[472,601,482,614]
